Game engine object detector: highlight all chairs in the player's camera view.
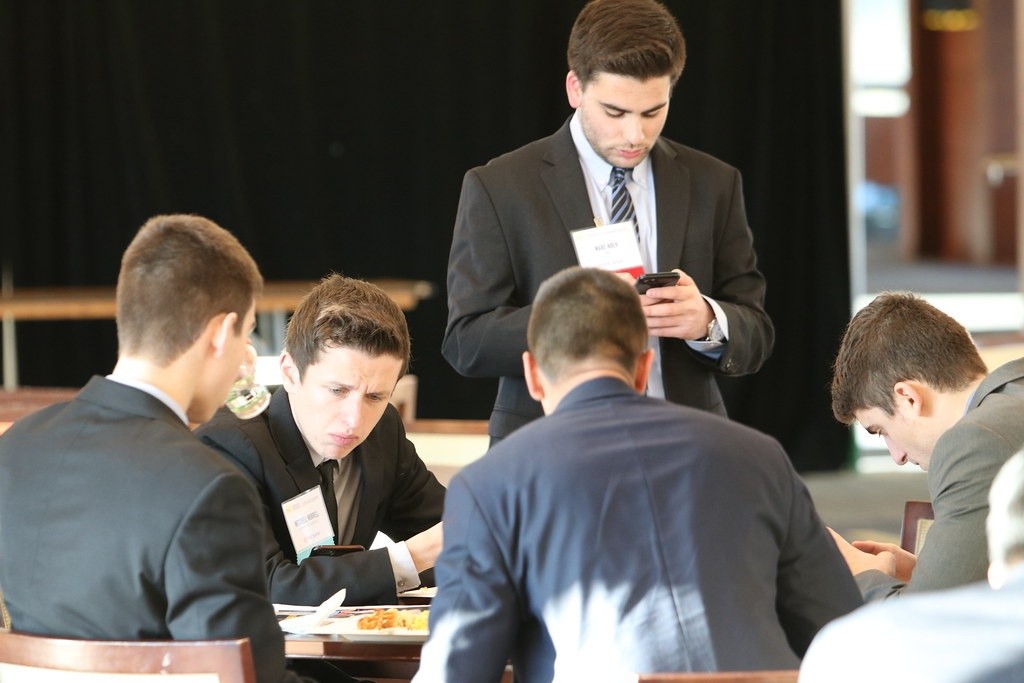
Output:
[0,631,256,683]
[901,500,937,558]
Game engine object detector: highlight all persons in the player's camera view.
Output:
[197,271,447,605]
[441,0,776,452]
[0,214,296,683]
[826,290,1024,601]
[405,267,864,682]
[797,449,1024,682]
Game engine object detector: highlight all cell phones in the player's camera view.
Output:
[310,545,366,557]
[635,272,680,295]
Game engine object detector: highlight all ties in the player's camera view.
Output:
[610,167,640,242]
[316,459,338,546]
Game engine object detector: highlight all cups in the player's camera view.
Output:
[224,361,271,420]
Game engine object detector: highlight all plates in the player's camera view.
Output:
[279,615,430,642]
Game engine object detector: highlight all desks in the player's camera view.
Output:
[0,280,431,392]
[279,601,512,683]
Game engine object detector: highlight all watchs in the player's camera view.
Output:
[702,317,724,343]
